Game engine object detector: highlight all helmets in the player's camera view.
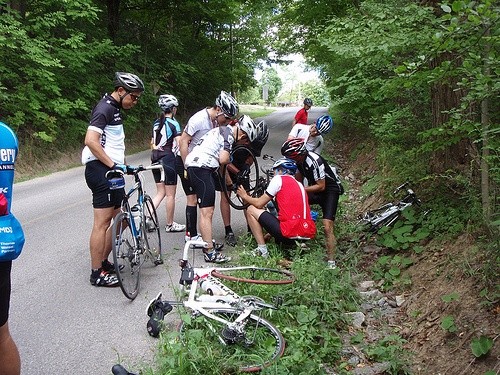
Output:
[254,120,270,148]
[113,71,145,92]
[216,90,240,118]
[281,137,306,158]
[316,115,333,134]
[303,98,312,107]
[236,115,258,142]
[273,158,297,173]
[158,93,179,110]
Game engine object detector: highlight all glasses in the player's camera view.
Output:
[223,113,233,120]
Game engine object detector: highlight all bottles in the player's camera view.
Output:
[196,295,231,303]
[199,280,225,295]
[131,204,142,231]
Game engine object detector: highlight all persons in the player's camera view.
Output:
[219,119,270,247]
[82,71,145,288]
[281,137,344,270]
[142,94,187,233]
[236,157,317,258]
[174,90,240,248]
[287,115,334,155]
[185,114,258,263]
[291,97,312,126]
[0,121,25,375]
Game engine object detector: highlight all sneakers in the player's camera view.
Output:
[327,261,339,272]
[244,231,254,240]
[145,217,157,232]
[102,259,125,273]
[90,270,119,286]
[202,241,224,252]
[203,252,232,264]
[165,222,186,232]
[224,233,237,246]
[184,234,216,249]
[239,248,270,260]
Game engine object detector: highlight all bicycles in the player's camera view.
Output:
[146,240,296,372]
[222,146,278,215]
[112,364,137,375]
[358,180,434,241]
[104,159,167,299]
